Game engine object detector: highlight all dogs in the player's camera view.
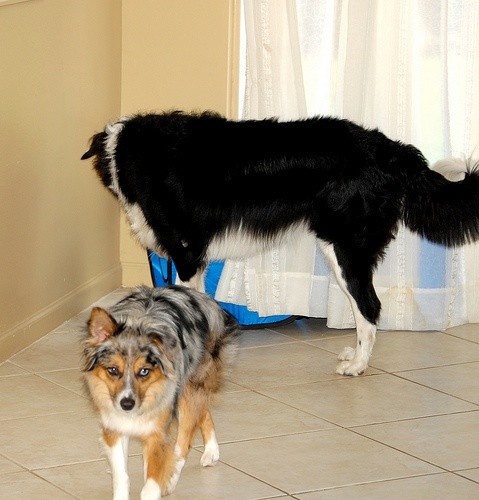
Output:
[78,283,238,500]
[78,110,479,379]
